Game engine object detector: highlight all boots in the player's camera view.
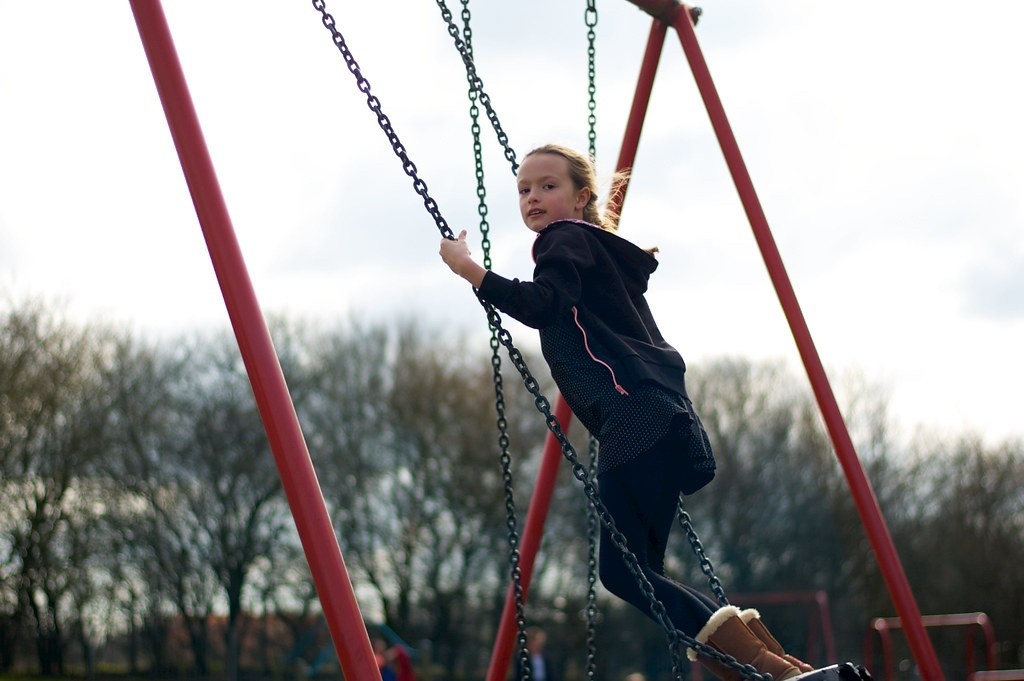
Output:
[740,609,814,674]
[687,605,801,680]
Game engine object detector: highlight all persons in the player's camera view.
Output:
[439,145,813,681]
[370,636,397,681]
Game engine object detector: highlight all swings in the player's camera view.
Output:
[309,0,870,681]
